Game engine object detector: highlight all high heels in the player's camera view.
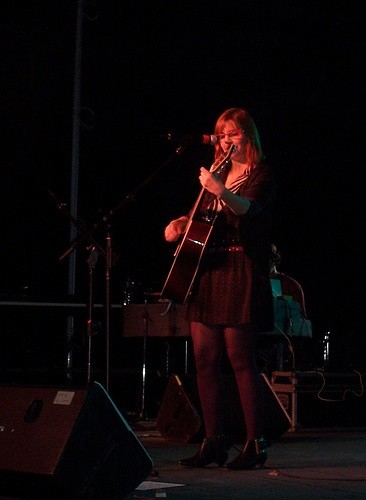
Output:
[225,437,268,471]
[179,434,229,468]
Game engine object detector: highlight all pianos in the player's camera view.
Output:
[119,275,314,341]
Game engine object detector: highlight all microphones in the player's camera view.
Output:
[167,133,217,144]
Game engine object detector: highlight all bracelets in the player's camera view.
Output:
[217,187,227,200]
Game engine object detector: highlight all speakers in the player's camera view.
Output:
[0,381,153,500]
[157,372,292,446]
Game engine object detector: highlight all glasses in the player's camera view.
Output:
[217,130,243,139]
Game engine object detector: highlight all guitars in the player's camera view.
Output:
[159,141,240,307]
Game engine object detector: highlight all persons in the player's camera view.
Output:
[164,107,275,470]
